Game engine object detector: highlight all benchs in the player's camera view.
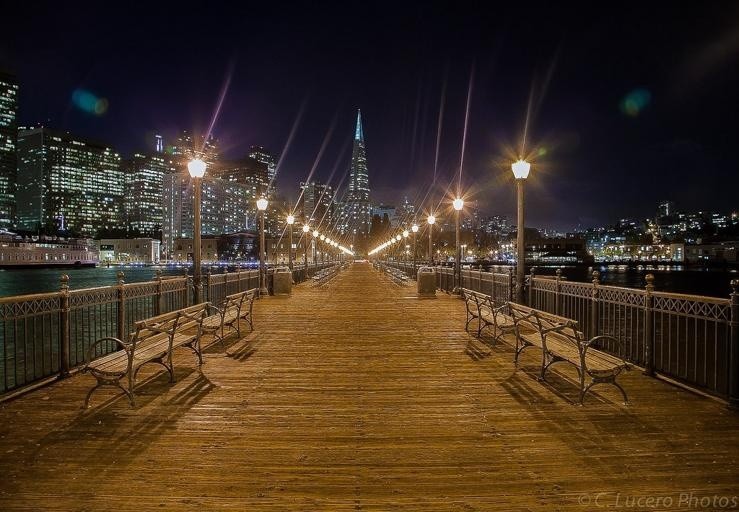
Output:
[462,286,519,343]
[505,299,629,406]
[78,302,213,409]
[189,285,258,352]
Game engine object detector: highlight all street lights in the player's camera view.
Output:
[511,153,532,305]
[187,152,207,320]
[412,221,419,280]
[313,227,409,277]
[452,191,464,294]
[303,223,310,279]
[256,194,268,296]
[287,210,295,284]
[426,211,436,266]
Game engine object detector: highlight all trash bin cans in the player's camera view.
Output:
[273,267,292,297]
[416,267,437,299]
